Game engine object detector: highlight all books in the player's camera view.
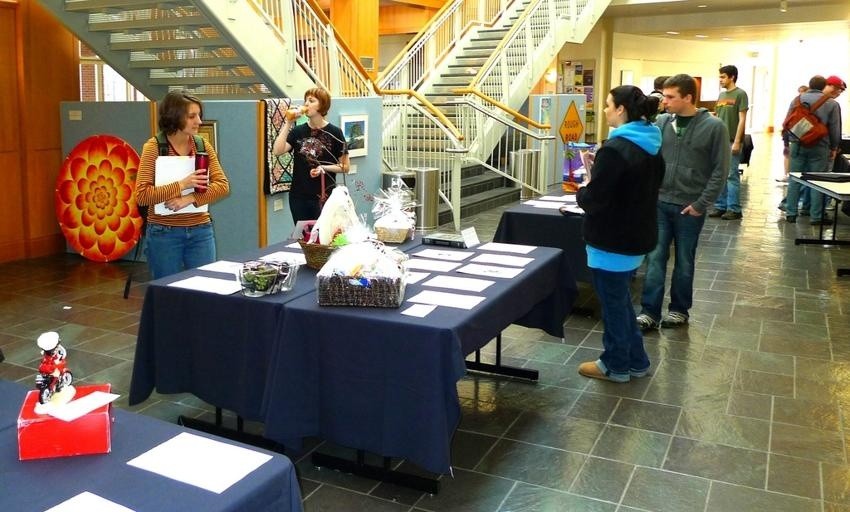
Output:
[421,232,468,248]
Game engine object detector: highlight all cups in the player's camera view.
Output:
[196,153,208,193]
[239,261,301,297]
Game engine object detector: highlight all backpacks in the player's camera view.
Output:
[136,130,206,221]
[782,93,835,147]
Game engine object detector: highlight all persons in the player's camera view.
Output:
[578,85,666,384]
[272,88,350,237]
[649,75,670,114]
[708,64,750,221]
[776,74,847,227]
[134,88,229,281]
[637,74,733,332]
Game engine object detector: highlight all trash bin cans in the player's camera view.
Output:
[510,149,541,201]
[383,168,439,231]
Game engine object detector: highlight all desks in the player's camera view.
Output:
[504,187,593,267]
[144,229,432,497]
[786,168,850,277]
[281,235,565,497]
[0,378,291,512]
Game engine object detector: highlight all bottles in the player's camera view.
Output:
[284,105,311,119]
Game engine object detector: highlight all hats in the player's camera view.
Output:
[824,75,846,90]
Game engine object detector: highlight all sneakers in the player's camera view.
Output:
[776,176,790,182]
[662,310,688,329]
[635,314,659,332]
[786,215,797,224]
[809,219,835,226]
[708,208,743,220]
[579,361,622,383]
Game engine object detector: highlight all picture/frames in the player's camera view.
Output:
[340,114,369,158]
